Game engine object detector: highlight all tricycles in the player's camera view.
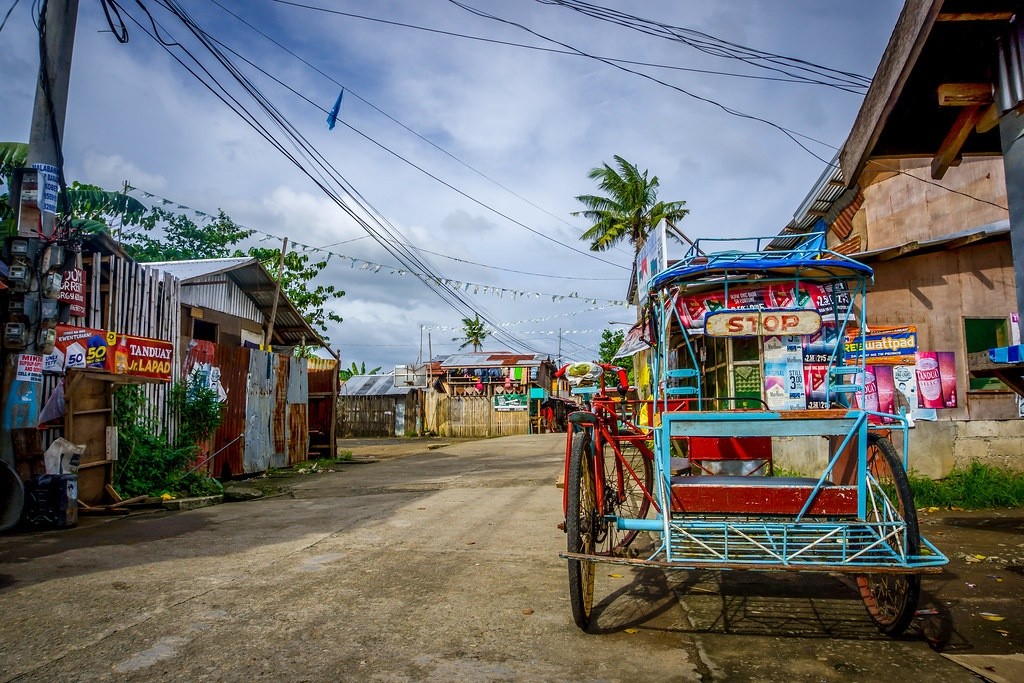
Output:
[552,231,951,637]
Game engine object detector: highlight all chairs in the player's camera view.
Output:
[686,434,773,477]
